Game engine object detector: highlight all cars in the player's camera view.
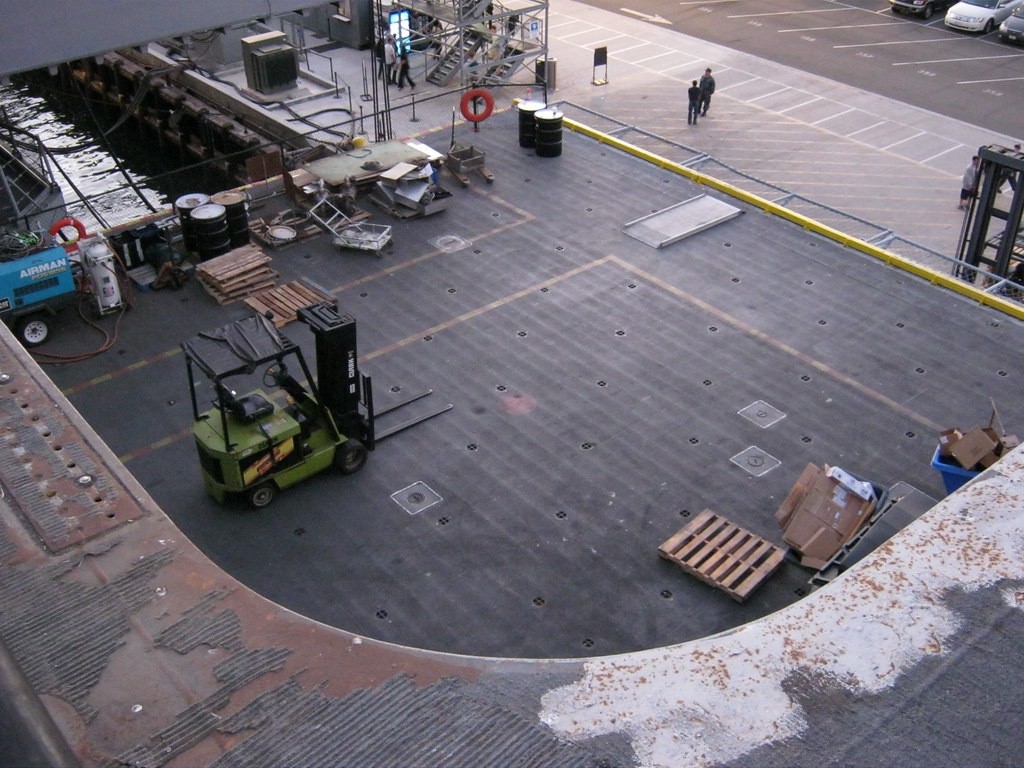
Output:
[999,3,1024,47]
[889,0,961,20]
[944,0,1023,34]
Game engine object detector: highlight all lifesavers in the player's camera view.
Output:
[457,86,496,123]
[49,215,88,253]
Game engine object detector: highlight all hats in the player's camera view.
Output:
[705,68,711,72]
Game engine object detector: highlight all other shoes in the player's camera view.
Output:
[693,110,707,117]
[688,120,697,125]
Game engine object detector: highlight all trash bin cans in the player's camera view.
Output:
[931,425,983,496]
[534,56,557,89]
[783,462,889,580]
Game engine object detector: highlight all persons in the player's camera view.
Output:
[375,38,385,79]
[959,144,1022,211]
[392,34,400,57]
[431,21,446,61]
[465,0,494,29]
[688,80,700,125]
[383,35,397,84]
[397,45,416,91]
[693,67,715,117]
[468,52,479,88]
[506,15,520,39]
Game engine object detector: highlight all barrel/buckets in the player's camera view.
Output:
[211,190,250,250]
[517,100,547,148]
[190,203,232,263]
[534,108,562,157]
[176,192,211,253]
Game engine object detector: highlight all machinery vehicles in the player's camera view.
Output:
[180,302,453,510]
